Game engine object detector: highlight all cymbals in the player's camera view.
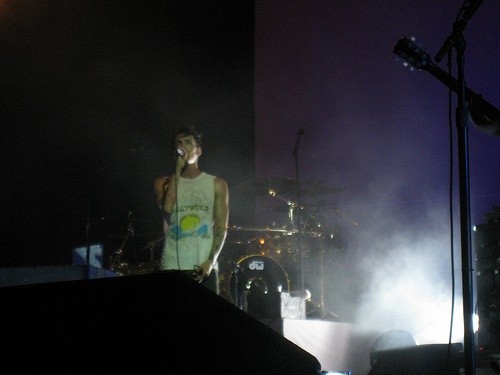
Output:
[296,187,347,196]
[236,176,302,198]
[275,203,316,211]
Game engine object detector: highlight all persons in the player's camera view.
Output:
[154,127,229,294]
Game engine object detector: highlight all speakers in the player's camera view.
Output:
[0,269,322,375]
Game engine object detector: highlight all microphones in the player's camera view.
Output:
[173,148,184,156]
[294,128,305,156]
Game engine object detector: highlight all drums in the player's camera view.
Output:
[228,253,291,307]
[278,219,316,258]
[218,239,250,271]
